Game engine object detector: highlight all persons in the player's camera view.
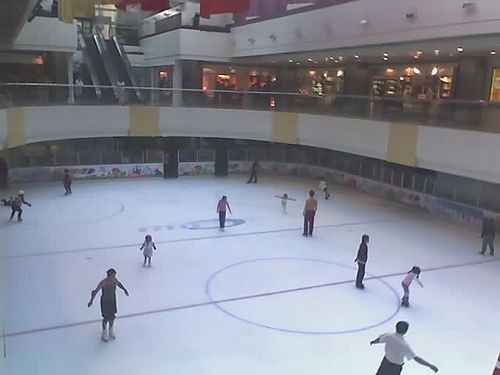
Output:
[216,157,496,308]
[88,268,130,342]
[75,76,125,105]
[63,169,72,196]
[140,234,157,268]
[0,157,31,222]
[37,74,52,105]
[370,321,439,375]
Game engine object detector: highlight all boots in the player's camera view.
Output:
[109,327,115,339]
[102,330,109,342]
